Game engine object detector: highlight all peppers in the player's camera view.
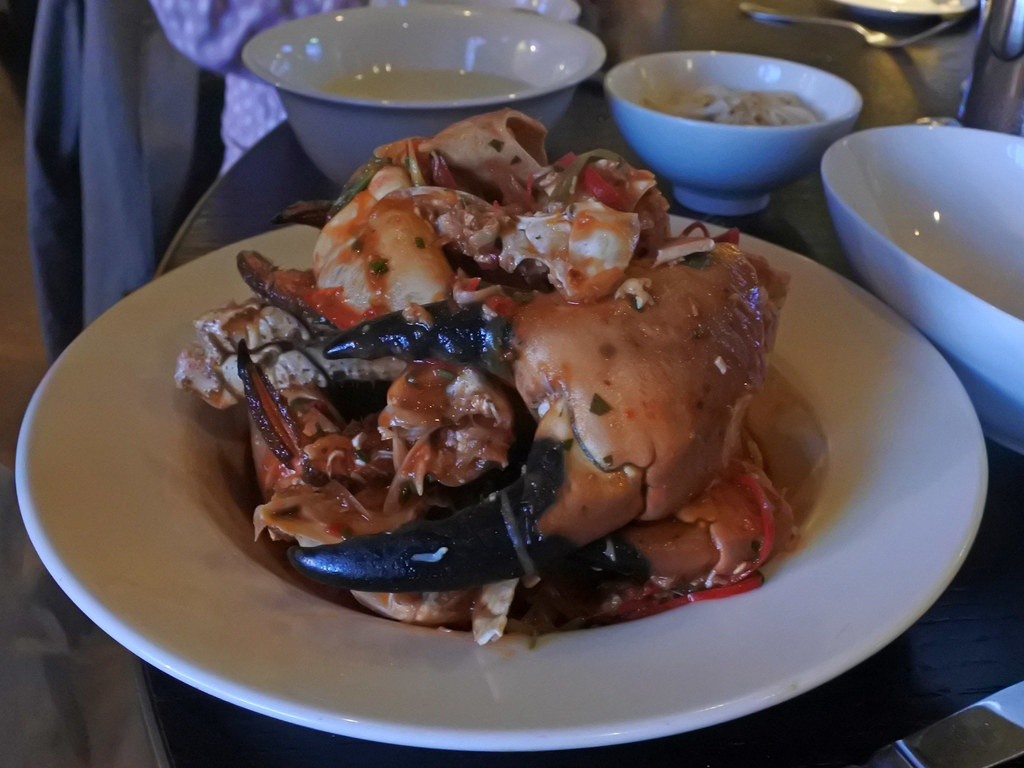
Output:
[559,151,624,212]
[739,473,773,577]
[633,568,763,617]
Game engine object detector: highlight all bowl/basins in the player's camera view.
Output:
[602,48,866,214]
[238,5,608,193]
[819,124,1024,455]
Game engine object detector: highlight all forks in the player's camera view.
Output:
[738,2,958,50]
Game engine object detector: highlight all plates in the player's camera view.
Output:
[835,0,981,24]
[366,1,582,28]
[16,212,991,753]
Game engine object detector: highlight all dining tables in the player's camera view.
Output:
[133,1,1024,768]
